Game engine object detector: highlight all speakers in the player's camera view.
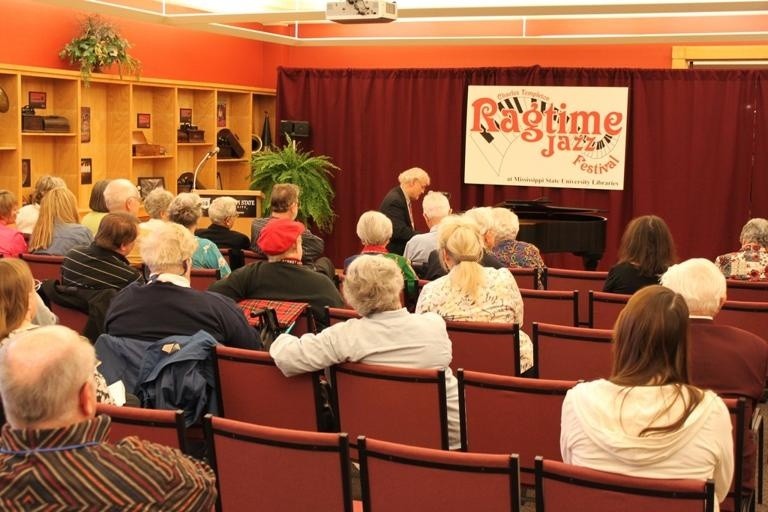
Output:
[277,119,312,139]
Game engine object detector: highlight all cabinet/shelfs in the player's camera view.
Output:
[0,63,277,228]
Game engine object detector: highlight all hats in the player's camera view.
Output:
[257,218,306,256]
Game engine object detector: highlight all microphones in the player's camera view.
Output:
[207,145,220,160]
[427,190,451,197]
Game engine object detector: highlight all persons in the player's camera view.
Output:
[81,180,110,236]
[0,191,27,259]
[560,284,735,512]
[29,176,67,239]
[250,183,325,264]
[267,253,462,451]
[356,210,419,304]
[378,167,430,257]
[603,215,675,293]
[481,206,547,290]
[415,222,534,375]
[715,218,768,280]
[0,258,33,341]
[195,196,250,270]
[103,178,143,268]
[29,187,93,256]
[168,192,232,280]
[144,187,172,225]
[105,220,266,351]
[29,273,58,324]
[61,214,146,291]
[403,190,455,263]
[657,257,768,404]
[0,322,217,511]
[206,220,344,334]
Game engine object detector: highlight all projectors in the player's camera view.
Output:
[324,2,398,25]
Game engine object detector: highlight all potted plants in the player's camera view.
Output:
[57,11,144,89]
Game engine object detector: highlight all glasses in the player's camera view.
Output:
[92,358,106,379]
[33,276,43,295]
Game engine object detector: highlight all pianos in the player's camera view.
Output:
[461,197,608,272]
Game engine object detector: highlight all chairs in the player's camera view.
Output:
[334,273,411,309]
[717,391,764,504]
[534,455,716,512]
[417,279,431,295]
[518,287,579,343]
[47,299,89,336]
[544,267,609,326]
[456,368,585,490]
[531,321,616,383]
[18,253,64,284]
[203,413,353,512]
[22,233,31,245]
[190,269,221,291]
[714,300,768,345]
[357,434,521,512]
[218,248,233,270]
[719,396,759,511]
[323,305,362,326]
[95,402,190,455]
[290,305,313,339]
[726,279,768,302]
[330,362,449,461]
[507,266,538,290]
[588,289,633,330]
[129,263,150,280]
[240,248,264,267]
[211,344,333,432]
[446,321,519,377]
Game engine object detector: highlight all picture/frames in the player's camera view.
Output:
[28,91,47,109]
[180,109,192,123]
[81,158,92,183]
[22,159,31,187]
[81,106,90,143]
[217,172,222,190]
[137,114,150,128]
[138,176,165,201]
